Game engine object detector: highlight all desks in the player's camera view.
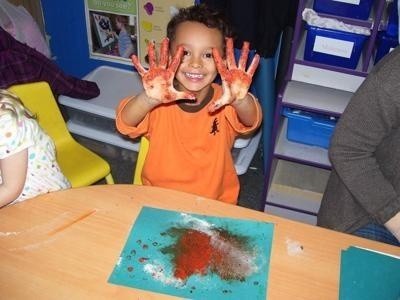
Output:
[0,185,400,300]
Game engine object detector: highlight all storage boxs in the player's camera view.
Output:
[302,0,398,71]
[280,108,338,150]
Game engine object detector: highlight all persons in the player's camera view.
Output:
[108,16,136,60]
[115,2,264,207]
[316,46,399,250]
[0,87,72,210]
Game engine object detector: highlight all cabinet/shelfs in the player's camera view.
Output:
[259,0,400,247]
